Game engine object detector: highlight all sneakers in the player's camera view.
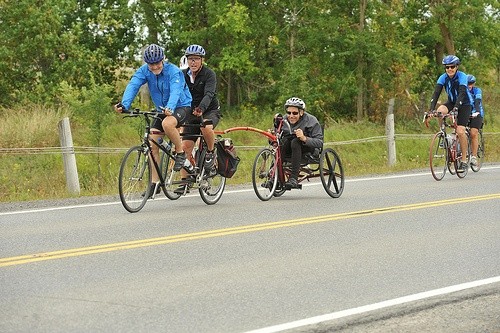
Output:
[457,162,468,172]
[141,182,162,197]
[205,149,217,169]
[440,141,444,148]
[174,150,188,171]
[174,183,190,194]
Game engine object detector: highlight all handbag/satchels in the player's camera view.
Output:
[217,138,241,179]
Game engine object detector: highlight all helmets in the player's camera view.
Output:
[284,97,306,110]
[466,73,475,84]
[145,44,164,63]
[442,55,459,66]
[184,44,206,57]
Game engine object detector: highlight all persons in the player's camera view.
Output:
[261,97,323,189]
[173,45,222,193]
[425,55,473,173]
[466,74,484,166]
[115,44,193,197]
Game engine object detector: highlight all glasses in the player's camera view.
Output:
[187,56,202,62]
[446,65,456,69]
[285,110,300,115]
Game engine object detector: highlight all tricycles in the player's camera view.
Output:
[252,113,345,202]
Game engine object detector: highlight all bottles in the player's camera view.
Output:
[157,137,171,151]
[448,135,453,148]
[172,151,194,171]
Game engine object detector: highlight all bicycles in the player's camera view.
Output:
[422,110,486,181]
[116,101,235,213]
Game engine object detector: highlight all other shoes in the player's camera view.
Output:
[261,180,280,189]
[284,178,299,189]
[469,156,477,165]
